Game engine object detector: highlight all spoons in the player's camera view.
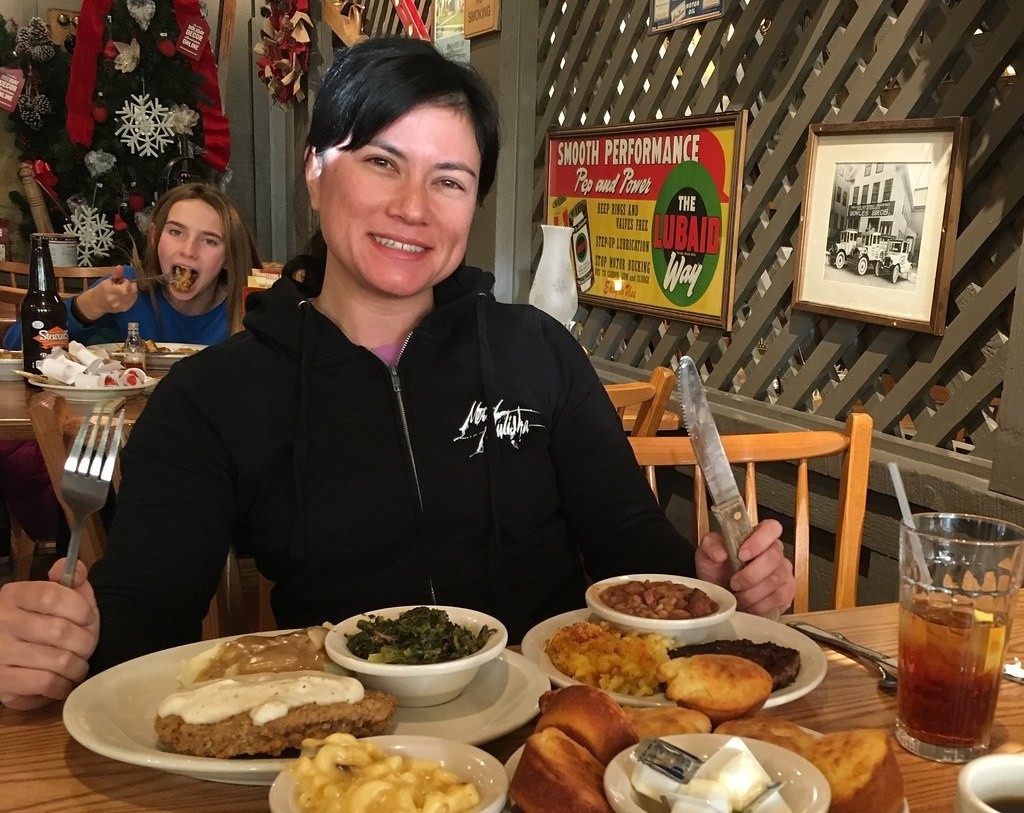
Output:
[830,632,898,690]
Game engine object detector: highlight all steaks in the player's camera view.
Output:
[662,638,801,694]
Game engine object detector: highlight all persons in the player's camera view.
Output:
[0,32,802,714]
[3,181,263,351]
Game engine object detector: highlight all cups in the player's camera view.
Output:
[956,752,1024,813]
[894,512,1024,762]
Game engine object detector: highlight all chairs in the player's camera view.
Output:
[601,364,874,612]
[0,259,133,326]
[25,386,276,644]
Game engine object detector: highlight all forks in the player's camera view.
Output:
[110,273,181,284]
[58,402,126,589]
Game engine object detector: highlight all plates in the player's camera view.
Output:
[27,375,161,405]
[503,724,910,813]
[86,342,210,371]
[61,627,552,787]
[521,608,828,710]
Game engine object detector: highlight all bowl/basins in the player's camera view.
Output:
[268,734,511,813]
[585,572,737,645]
[323,604,508,708]
[603,732,832,813]
[0,350,24,382]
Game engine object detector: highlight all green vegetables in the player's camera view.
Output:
[344,606,498,664]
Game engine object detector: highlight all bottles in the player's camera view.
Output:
[124,322,146,374]
[19,232,69,390]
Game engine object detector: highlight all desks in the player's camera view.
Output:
[0,364,170,440]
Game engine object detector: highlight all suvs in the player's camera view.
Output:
[827,229,860,269]
[846,230,889,276]
[875,238,913,283]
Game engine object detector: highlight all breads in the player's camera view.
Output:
[509,652,904,813]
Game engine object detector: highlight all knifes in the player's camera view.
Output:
[786,619,898,671]
[678,356,754,572]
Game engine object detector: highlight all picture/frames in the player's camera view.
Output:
[648,0,725,34]
[538,110,747,332]
[792,114,970,334]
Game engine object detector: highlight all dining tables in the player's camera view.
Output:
[0,574,1024,813]
